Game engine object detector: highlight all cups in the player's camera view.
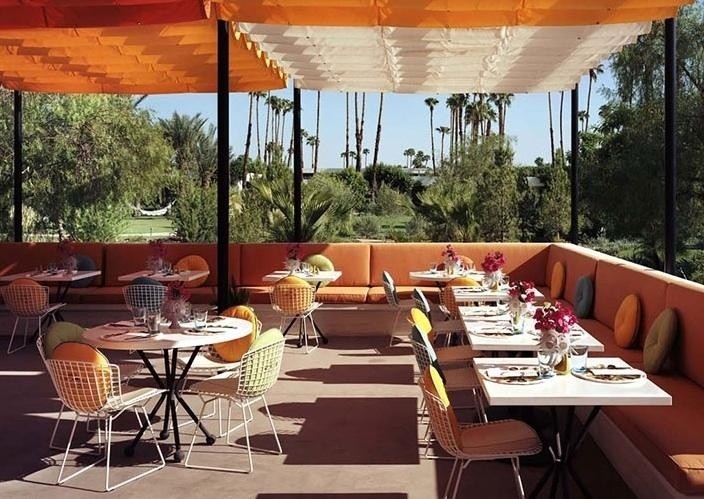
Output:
[537,349,557,379]
[163,262,172,272]
[148,311,161,334]
[430,262,438,272]
[469,264,475,272]
[303,263,311,276]
[193,306,209,329]
[570,344,587,372]
[131,306,147,325]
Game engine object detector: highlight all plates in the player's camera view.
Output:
[99,331,154,343]
[477,363,551,385]
[471,326,520,338]
[570,364,647,383]
